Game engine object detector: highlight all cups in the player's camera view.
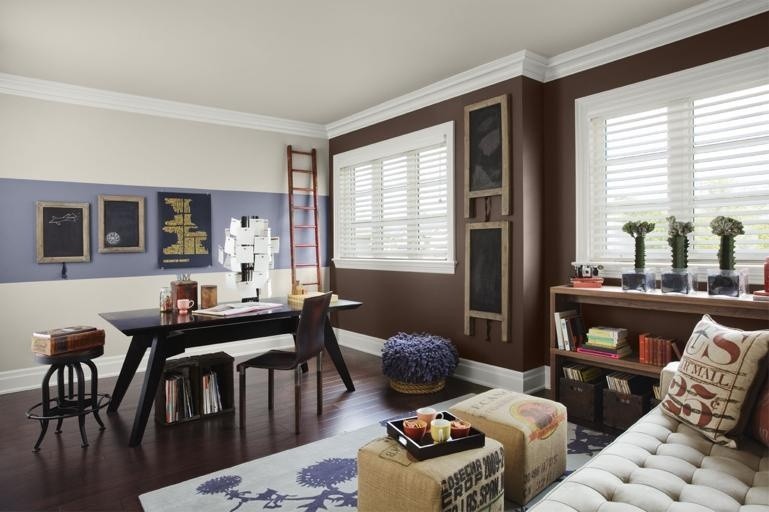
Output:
[177,315,194,324]
[450,420,471,439]
[430,419,452,446]
[403,419,427,441]
[176,298,194,314]
[182,271,190,281]
[416,406,445,432]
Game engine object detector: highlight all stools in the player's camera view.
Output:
[448,386,568,506]
[23,345,114,452]
[357,437,506,512]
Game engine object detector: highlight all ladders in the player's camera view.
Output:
[287,144,323,292]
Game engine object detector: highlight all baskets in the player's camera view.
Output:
[389,374,446,393]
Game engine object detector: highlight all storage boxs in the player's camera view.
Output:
[31,327,105,356]
[601,387,654,429]
[557,375,608,424]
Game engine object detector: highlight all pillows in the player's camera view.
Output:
[658,311,767,450]
[746,375,768,452]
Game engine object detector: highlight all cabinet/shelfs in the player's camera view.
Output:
[549,282,769,403]
[154,352,235,429]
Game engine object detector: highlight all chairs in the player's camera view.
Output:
[235,290,335,436]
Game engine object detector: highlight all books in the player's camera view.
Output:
[31,328,105,356]
[163,368,224,424]
[288,292,339,304]
[753,290,768,300]
[555,309,683,400]
[569,277,604,288]
[192,302,283,316]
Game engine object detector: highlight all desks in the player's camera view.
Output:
[97,295,365,449]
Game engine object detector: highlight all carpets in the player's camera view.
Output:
[134,390,623,512]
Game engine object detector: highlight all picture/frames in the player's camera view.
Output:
[98,194,146,255]
[36,200,91,264]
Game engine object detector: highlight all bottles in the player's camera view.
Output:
[159,312,171,325]
[159,287,175,313]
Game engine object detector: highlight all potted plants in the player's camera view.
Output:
[619,219,659,293]
[660,216,699,295]
[707,216,750,298]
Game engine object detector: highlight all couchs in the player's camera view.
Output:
[512,359,769,512]
